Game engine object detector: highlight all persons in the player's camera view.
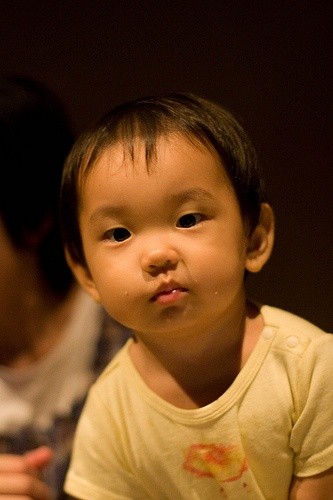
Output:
[59,85,333,500]
[0,71,136,500]
